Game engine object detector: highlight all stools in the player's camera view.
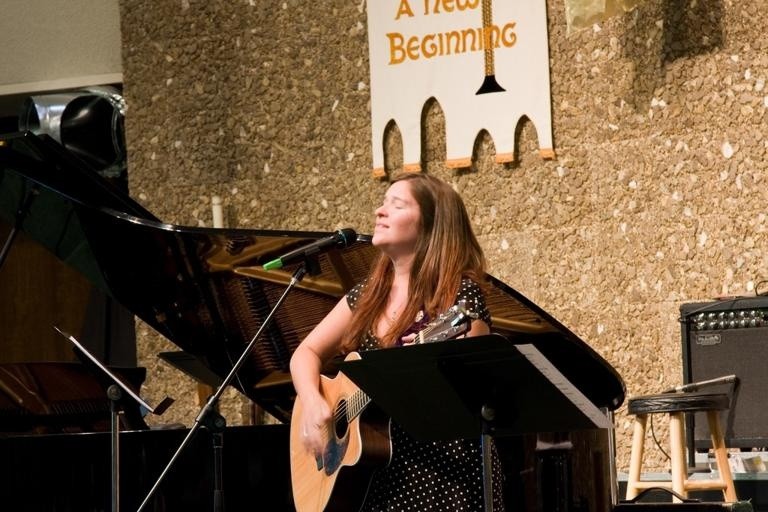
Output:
[626,392,740,502]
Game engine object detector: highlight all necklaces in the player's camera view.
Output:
[384,296,405,326]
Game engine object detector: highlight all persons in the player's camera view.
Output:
[290,168,507,512]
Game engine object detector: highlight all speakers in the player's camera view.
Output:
[681,296,767,448]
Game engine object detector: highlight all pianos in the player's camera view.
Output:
[0,132,627,510]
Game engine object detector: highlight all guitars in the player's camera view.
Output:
[289,303,476,512]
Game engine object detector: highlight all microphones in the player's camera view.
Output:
[263,228,356,271]
